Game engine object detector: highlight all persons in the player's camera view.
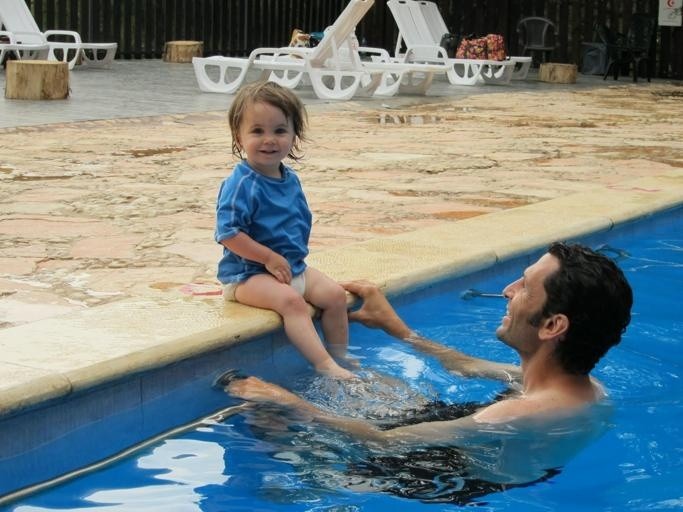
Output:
[212,79,378,388]
[218,239,634,512]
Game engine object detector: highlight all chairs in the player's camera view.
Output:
[516,16,557,62]
[596,24,658,83]
[0,0,118,71]
[192,0,532,101]
